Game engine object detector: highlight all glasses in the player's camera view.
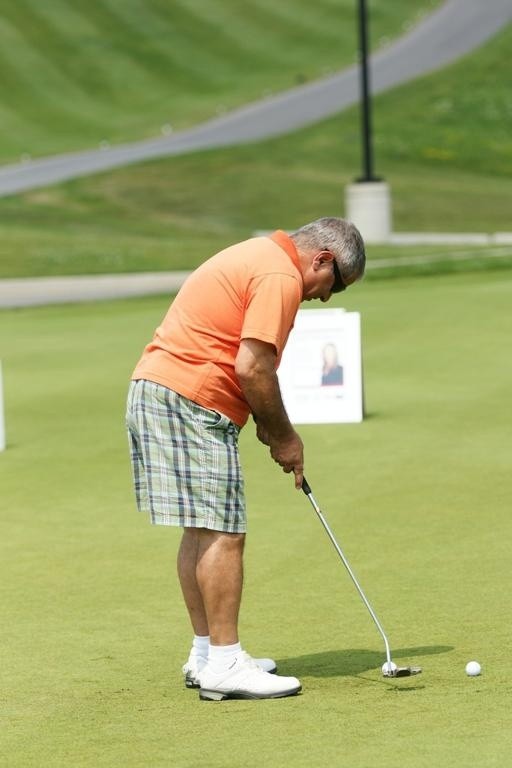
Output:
[325,249,347,294]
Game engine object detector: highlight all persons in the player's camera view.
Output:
[122,215,367,705]
[317,343,344,389]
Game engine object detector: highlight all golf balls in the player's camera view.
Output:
[465,662,481,676]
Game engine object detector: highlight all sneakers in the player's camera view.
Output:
[181,657,303,701]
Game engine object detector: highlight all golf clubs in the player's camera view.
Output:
[253,412,421,678]
